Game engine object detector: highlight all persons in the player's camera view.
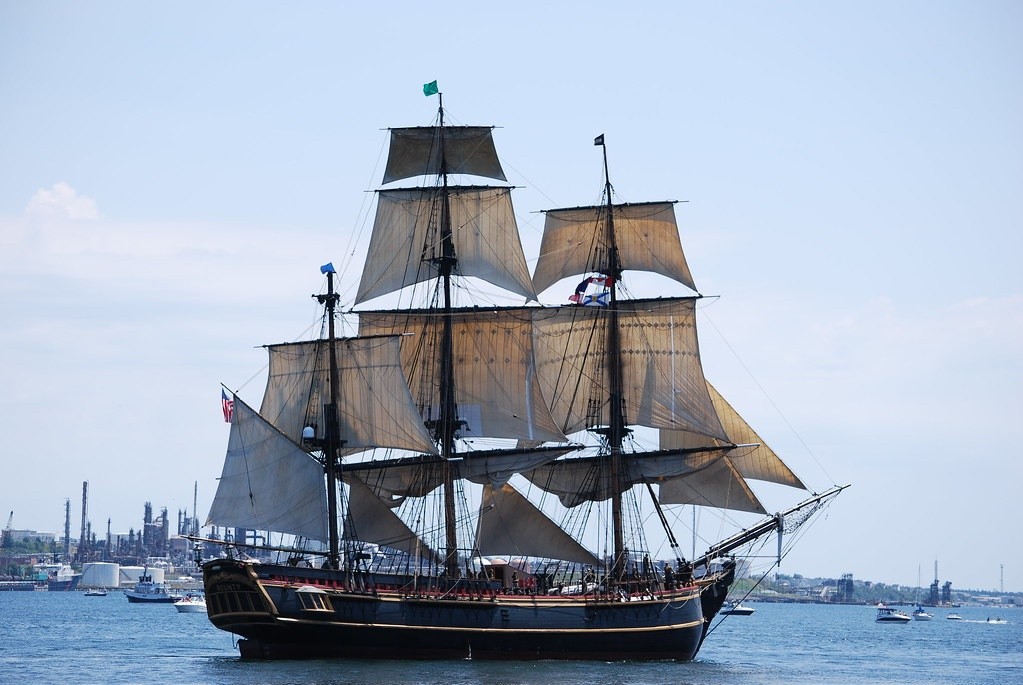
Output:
[511,570,521,595]
[524,557,694,597]
[388,564,396,573]
[288,554,343,572]
[439,567,496,580]
[643,553,650,576]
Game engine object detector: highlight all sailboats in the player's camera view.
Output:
[185,79,858,664]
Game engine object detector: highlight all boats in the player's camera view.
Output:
[0,565,72,592]
[173,593,207,612]
[718,596,755,616]
[122,564,182,602]
[946,611,962,620]
[913,564,935,620]
[67,572,82,591]
[84,583,108,595]
[875,606,911,624]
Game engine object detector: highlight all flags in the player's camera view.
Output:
[594,134,604,146]
[320,262,336,274]
[423,80,439,97]
[221,386,235,423]
[569,275,613,309]
